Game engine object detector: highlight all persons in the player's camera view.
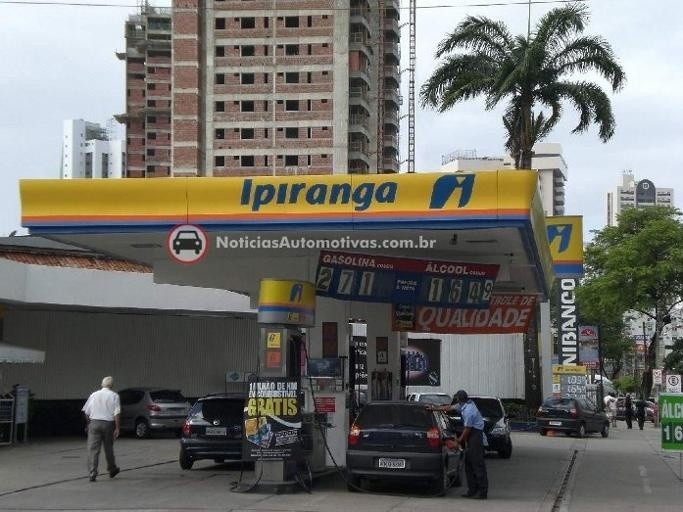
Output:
[424,390,488,499]
[81,376,122,482]
[603,392,648,430]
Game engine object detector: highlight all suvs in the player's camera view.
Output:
[534,395,609,438]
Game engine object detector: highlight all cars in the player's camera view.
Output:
[344,399,464,498]
[612,395,655,424]
[178,392,247,471]
[343,386,512,462]
[78,386,192,441]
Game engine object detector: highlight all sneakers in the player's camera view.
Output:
[89,474,98,481]
[461,489,489,499]
[109,467,120,477]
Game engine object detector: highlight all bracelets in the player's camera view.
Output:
[456,439,461,444]
[431,405,434,411]
